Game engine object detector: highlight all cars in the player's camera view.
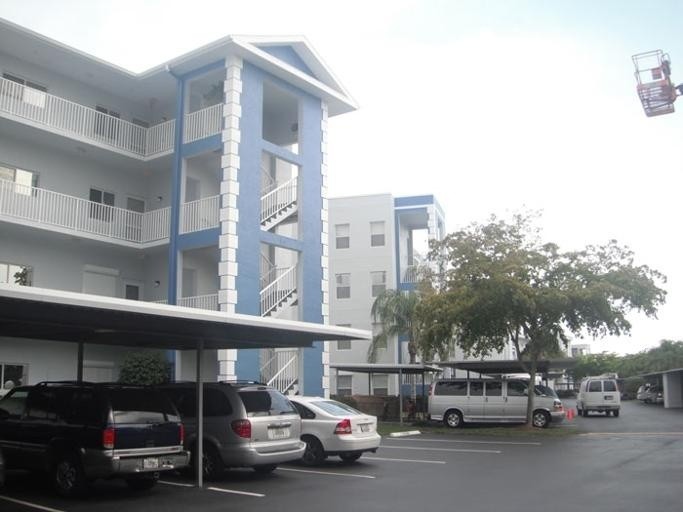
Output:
[284,394,381,466]
[636,385,667,404]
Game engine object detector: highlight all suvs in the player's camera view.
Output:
[0,380,191,495]
[140,380,308,480]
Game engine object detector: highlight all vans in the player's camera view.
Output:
[428,379,564,429]
[577,376,620,417]
